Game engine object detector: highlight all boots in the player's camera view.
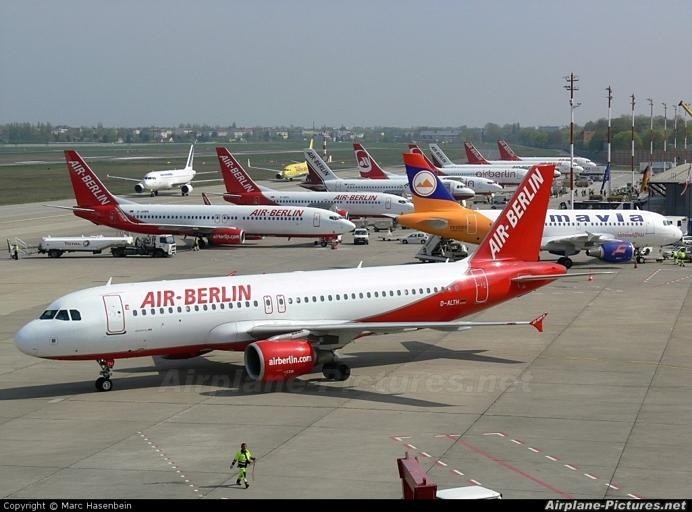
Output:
[245,482,249,488]
[237,479,241,485]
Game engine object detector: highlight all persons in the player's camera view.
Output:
[670,249,679,265]
[229,441,257,490]
[678,249,686,267]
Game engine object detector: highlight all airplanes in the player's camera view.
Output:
[40,139,683,268]
[13,167,615,391]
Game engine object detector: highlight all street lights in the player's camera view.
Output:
[571,103,582,209]
